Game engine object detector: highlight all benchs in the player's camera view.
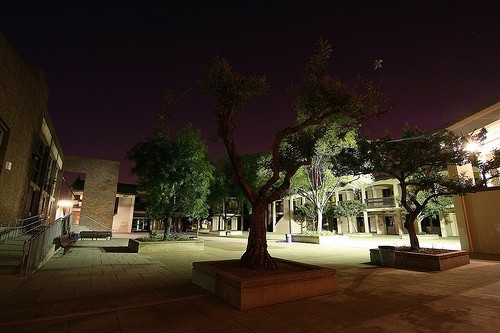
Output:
[58,231,81,255]
[80,231,113,240]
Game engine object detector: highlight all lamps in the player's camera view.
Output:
[4,158,12,171]
